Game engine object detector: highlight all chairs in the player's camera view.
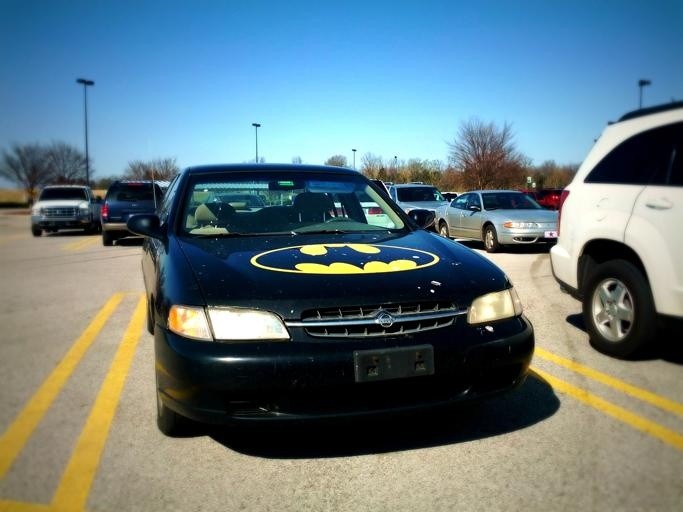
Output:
[188,202,236,234]
[284,193,333,229]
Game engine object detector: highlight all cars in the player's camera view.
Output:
[30,183,102,237]
[126,162,536,438]
[548,100,682,361]
[326,177,561,254]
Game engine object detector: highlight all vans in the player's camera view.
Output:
[99,180,164,247]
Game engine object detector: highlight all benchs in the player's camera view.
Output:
[188,202,293,227]
